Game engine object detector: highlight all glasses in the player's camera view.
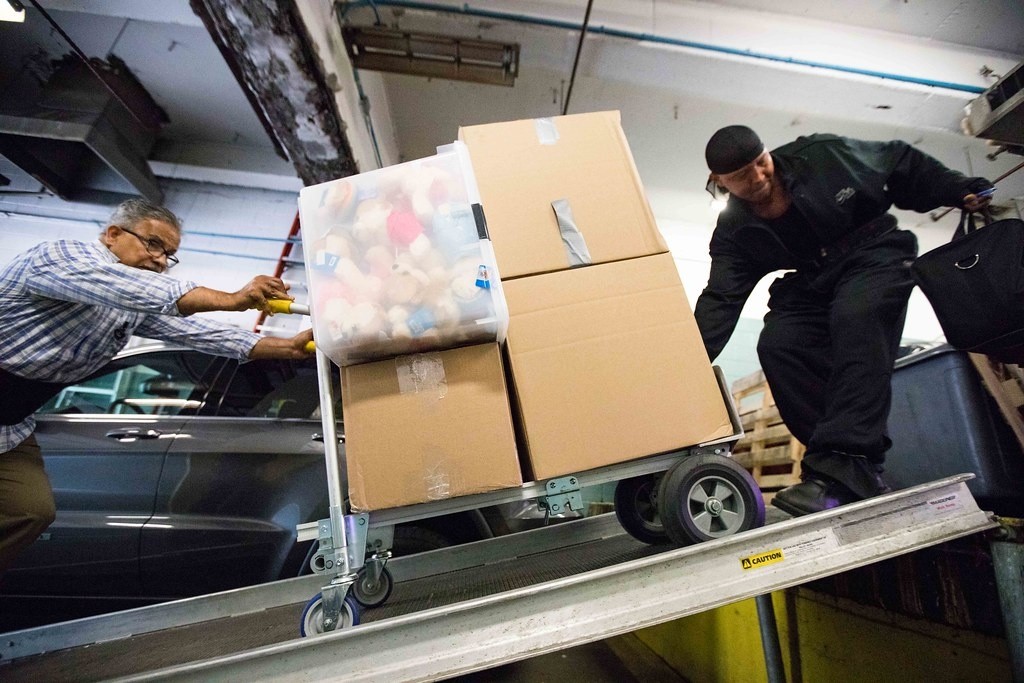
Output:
[122,227,179,268]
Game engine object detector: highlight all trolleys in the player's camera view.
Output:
[251,299,764,644]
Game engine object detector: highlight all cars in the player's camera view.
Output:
[0,341,584,628]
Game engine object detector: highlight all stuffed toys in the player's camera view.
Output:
[302,154,496,366]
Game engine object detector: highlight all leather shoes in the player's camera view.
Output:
[772,475,864,517]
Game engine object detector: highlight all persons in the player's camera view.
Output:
[0,199,313,572]
[694,125,996,517]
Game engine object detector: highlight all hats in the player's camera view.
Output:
[706,125,764,174]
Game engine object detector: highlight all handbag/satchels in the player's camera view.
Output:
[908,207,1023,366]
